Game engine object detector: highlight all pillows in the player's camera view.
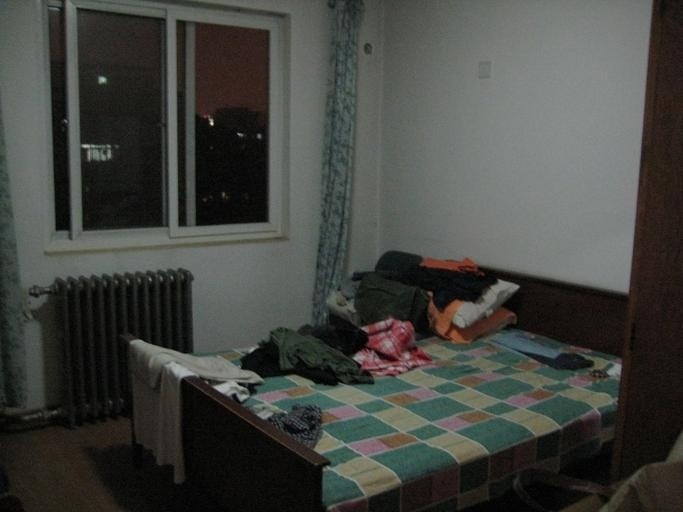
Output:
[426,277,520,344]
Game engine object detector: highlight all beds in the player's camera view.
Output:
[120,250,630,512]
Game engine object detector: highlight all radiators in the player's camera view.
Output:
[29,267,193,430]
